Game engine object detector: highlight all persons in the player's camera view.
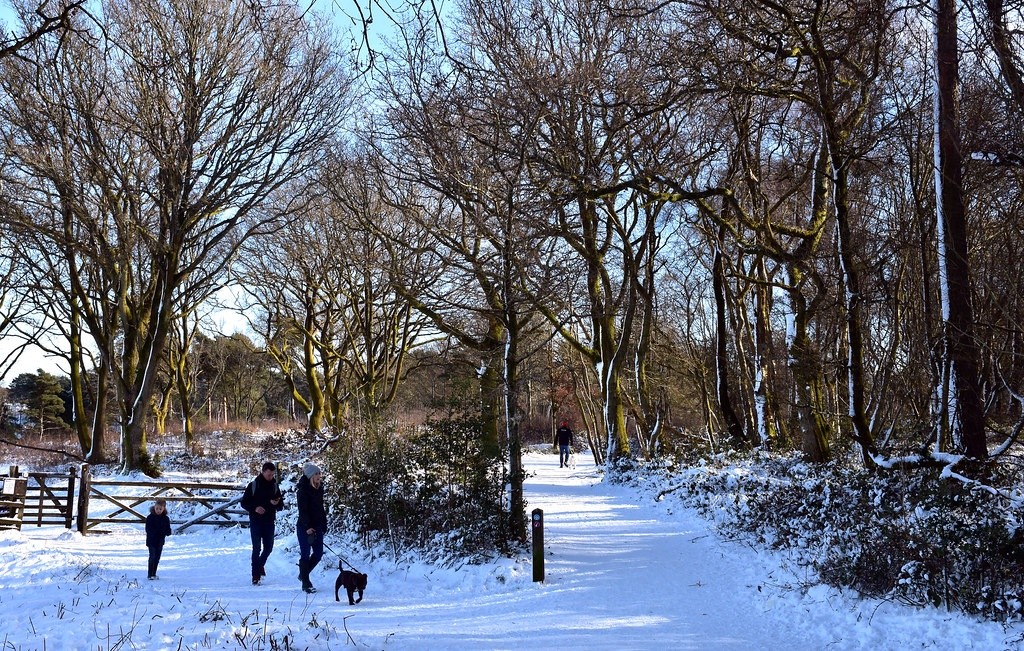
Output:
[241,463,283,584]
[554,420,573,468]
[296,463,328,593]
[146,498,172,580]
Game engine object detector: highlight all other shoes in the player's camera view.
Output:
[564,462,568,467]
[560,465,563,468]
[149,576,155,580]
[261,567,265,575]
[253,576,258,583]
[303,587,317,593]
[155,576,158,580]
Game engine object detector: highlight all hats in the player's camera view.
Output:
[304,463,320,479]
[562,421,568,426]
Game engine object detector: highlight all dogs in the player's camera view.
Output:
[335,560,367,606]
